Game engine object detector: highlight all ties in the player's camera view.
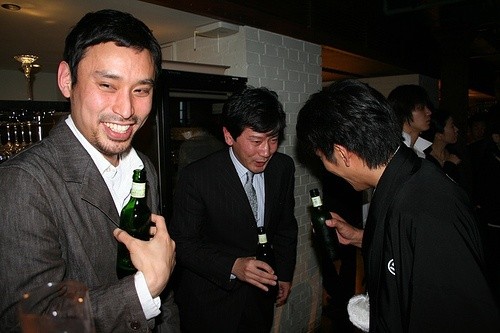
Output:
[244,171,257,222]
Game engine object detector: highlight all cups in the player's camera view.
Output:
[19,281,95,333]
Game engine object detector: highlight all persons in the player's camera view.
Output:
[296,81,500,333]
[0,9,177,333]
[388,84,500,284]
[169,85,299,333]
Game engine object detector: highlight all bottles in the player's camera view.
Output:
[256,226,280,299]
[116,169,152,279]
[307,188,340,263]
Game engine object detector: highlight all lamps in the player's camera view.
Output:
[14,55,40,101]
